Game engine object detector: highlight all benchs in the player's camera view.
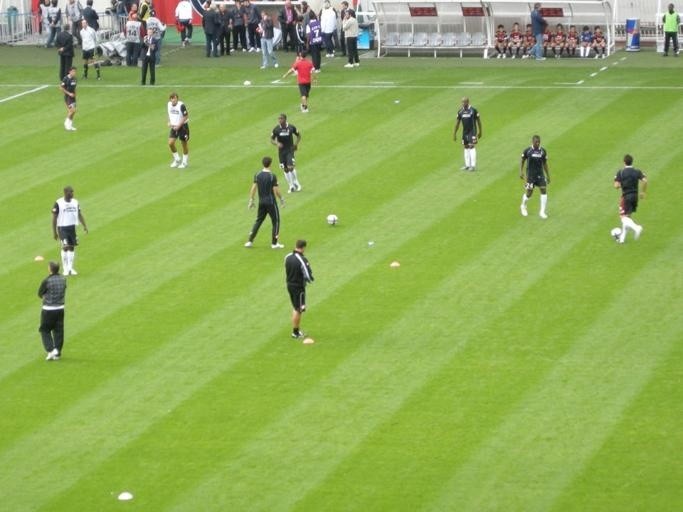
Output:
[490,44,608,59]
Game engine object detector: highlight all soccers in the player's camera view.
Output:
[610,227,622,238]
[327,214,338,226]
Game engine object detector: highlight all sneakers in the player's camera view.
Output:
[292,331,306,338]
[70,269,77,275]
[46,349,58,360]
[462,165,642,243]
[63,269,69,275]
[171,159,301,248]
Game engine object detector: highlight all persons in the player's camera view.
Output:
[166,93,190,169]
[244,156,286,249]
[59,67,78,130]
[51,187,87,275]
[270,113,302,194]
[202,0,360,72]
[174,0,195,48]
[37,0,168,85]
[282,50,318,113]
[493,22,607,60]
[453,96,481,172]
[662,4,680,57]
[523,2,548,60]
[520,135,550,219]
[38,261,66,360]
[614,154,648,243]
[283,240,315,343]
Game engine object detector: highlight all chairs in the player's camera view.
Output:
[382,32,487,59]
